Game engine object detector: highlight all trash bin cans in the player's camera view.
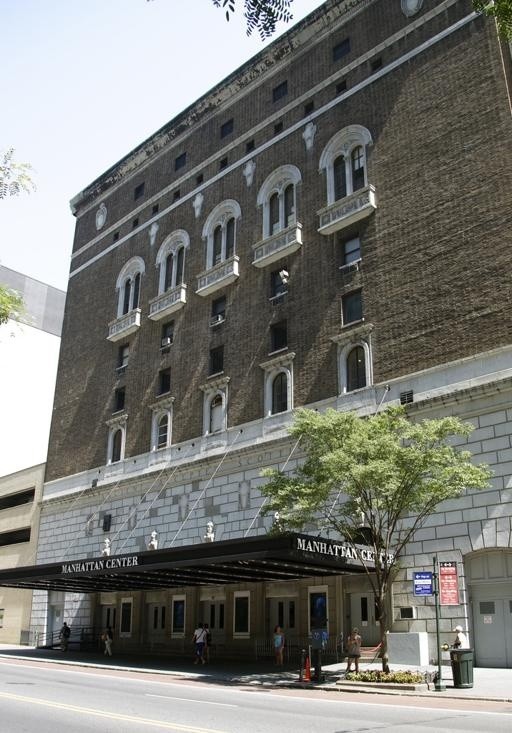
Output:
[450,648,474,688]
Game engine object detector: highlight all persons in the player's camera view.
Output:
[60,622,71,652]
[103,625,113,657]
[191,622,213,665]
[204,520,215,543]
[147,530,158,550]
[101,538,110,556]
[273,625,286,666]
[453,625,469,649]
[345,627,362,673]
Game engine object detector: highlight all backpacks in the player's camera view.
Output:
[63,627,70,638]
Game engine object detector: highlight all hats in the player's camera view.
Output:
[453,625,463,632]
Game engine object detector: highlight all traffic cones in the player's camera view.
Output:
[304,656,311,679]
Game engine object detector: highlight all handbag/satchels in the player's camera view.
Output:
[102,635,106,641]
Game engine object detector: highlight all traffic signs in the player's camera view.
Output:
[412,569,436,598]
[433,558,463,609]
